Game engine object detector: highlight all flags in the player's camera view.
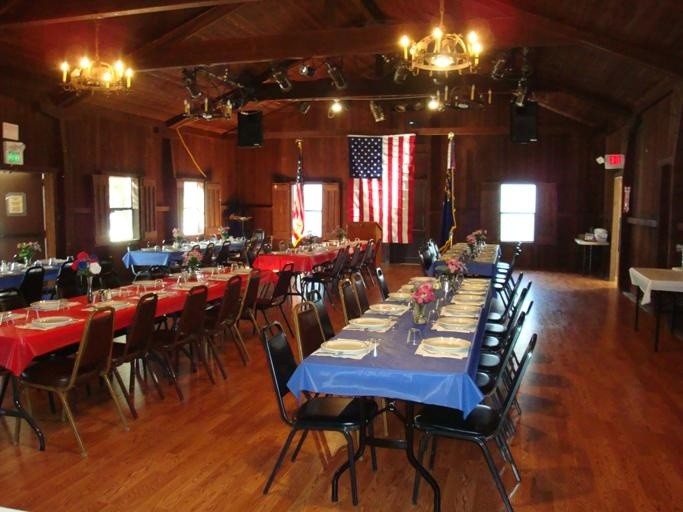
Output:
[439,139,457,257]
[292,148,304,248]
[347,133,416,244]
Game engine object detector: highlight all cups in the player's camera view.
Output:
[368,337,384,358]
[138,233,249,252]
[405,326,422,347]
[276,235,360,254]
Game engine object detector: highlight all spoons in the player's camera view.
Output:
[315,350,343,357]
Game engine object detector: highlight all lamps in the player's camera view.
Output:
[180,47,538,122]
[61,18,133,95]
[399,0,481,78]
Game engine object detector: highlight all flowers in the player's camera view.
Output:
[171,227,183,238]
[218,226,230,233]
[221,234,228,241]
[474,229,487,238]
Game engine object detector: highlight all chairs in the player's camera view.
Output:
[263,234,537,511]
[1,240,388,512]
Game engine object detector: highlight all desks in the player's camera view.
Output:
[628,267,683,353]
[574,238,610,278]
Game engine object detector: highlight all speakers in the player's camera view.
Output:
[510,102,537,144]
[238,111,263,146]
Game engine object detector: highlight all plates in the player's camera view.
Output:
[422,336,471,353]
[322,339,367,352]
[346,275,489,333]
[440,238,498,264]
[0,256,251,329]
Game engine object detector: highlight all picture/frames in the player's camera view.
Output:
[5,192,27,218]
